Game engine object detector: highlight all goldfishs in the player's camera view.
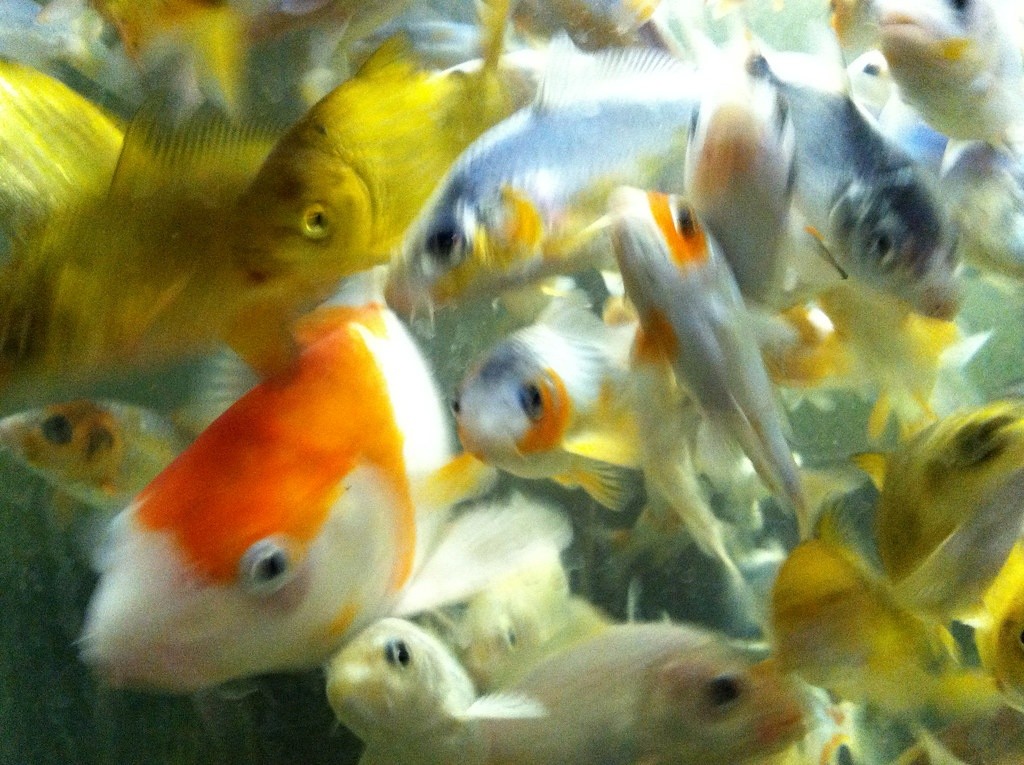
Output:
[323,533,1023,764]
[395,0,1024,537]
[2,3,452,762]
